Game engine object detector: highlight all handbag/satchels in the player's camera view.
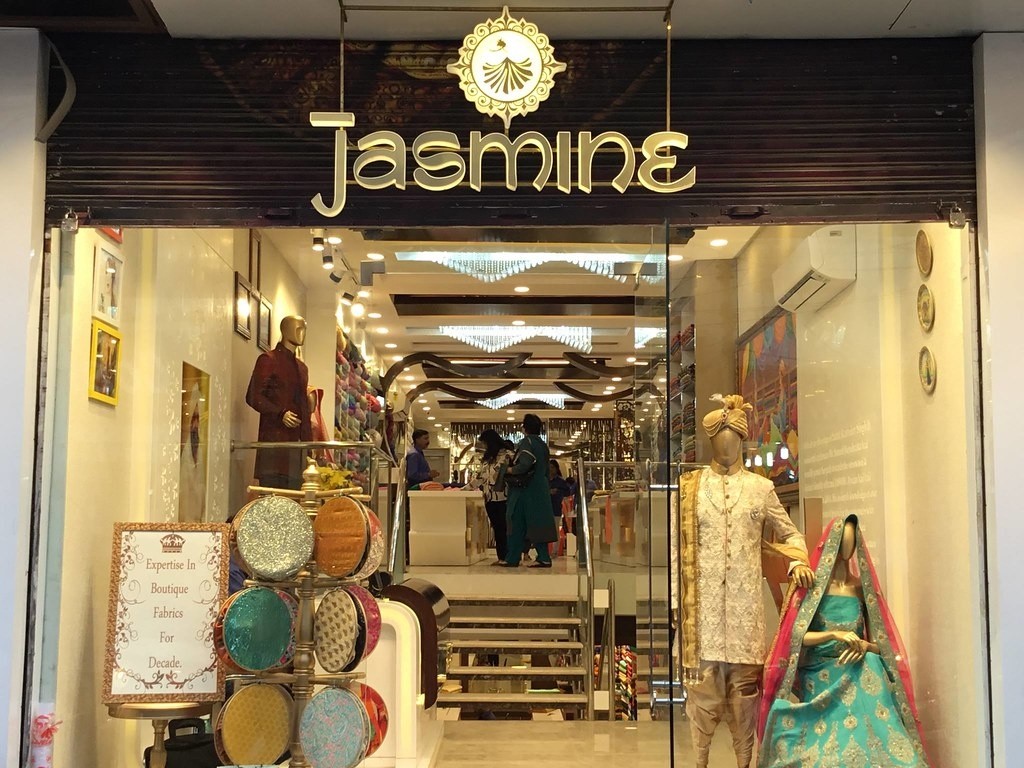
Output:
[504,457,536,489]
[492,462,508,489]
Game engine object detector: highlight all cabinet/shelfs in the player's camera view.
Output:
[668,258,737,500]
[335,325,370,510]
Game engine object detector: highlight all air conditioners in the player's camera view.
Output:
[772,224,856,314]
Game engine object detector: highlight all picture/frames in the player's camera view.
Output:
[919,345,936,395]
[257,294,272,353]
[916,283,934,332]
[88,320,121,405]
[100,227,124,243]
[101,522,231,704]
[234,271,252,340]
[93,240,124,328]
[914,229,933,276]
[249,228,262,300]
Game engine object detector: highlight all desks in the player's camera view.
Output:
[408,489,490,567]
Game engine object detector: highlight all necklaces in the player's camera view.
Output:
[704,467,745,515]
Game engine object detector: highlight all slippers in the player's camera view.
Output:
[498,558,518,567]
[527,559,551,567]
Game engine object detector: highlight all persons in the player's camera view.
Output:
[670,393,819,767]
[245,316,311,505]
[564,468,598,517]
[306,385,337,468]
[493,413,558,568]
[754,513,936,768]
[405,429,440,565]
[549,459,570,559]
[459,429,516,566]
[180,381,207,523]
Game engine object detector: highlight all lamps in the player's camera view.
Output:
[327,264,348,287]
[426,251,629,283]
[322,247,336,269]
[340,283,358,306]
[353,298,368,317]
[310,228,325,251]
[440,326,593,354]
[474,394,566,410]
